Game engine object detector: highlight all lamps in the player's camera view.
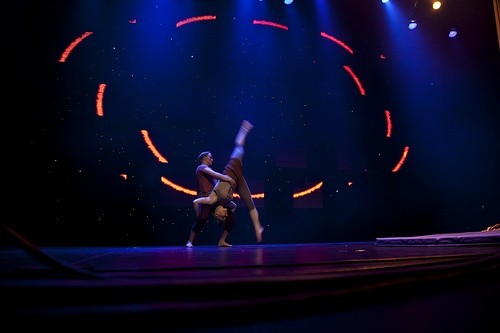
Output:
[409,19,417,30]
[449,27,457,37]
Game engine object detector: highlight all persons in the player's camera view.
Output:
[185,151,237,248]
[193,119,264,243]
